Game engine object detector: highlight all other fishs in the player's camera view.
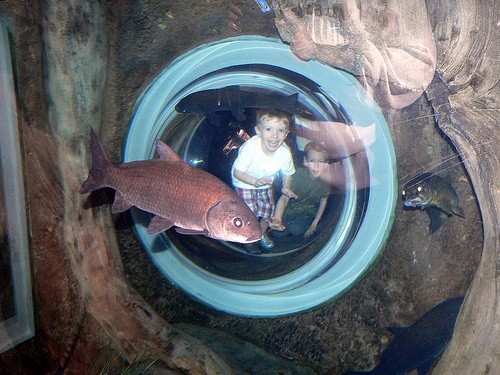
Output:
[78,125,264,244]
[175,85,299,126]
[401,173,465,235]
[343,296,464,375]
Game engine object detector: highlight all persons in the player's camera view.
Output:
[270,140,330,239]
[230,109,297,255]
